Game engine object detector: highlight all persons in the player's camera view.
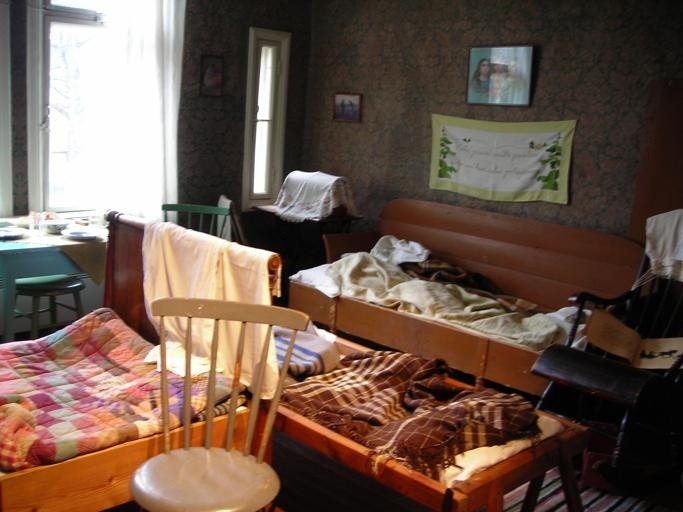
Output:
[471,59,492,93]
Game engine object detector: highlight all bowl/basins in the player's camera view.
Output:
[40,221,69,234]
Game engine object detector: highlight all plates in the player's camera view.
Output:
[0,226,26,239]
[61,229,97,240]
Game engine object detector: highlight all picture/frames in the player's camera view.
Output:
[331,92,363,124]
[464,43,536,108]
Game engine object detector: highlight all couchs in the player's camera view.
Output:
[322,196,646,398]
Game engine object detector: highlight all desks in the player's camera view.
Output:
[0,211,107,343]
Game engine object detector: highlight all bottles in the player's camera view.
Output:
[28,210,40,232]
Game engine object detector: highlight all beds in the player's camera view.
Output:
[0,208,282,511]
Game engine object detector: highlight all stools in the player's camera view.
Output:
[530,341,651,478]
[13,274,86,341]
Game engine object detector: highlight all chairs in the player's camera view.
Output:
[565,261,682,423]
[160,194,250,246]
[129,295,309,512]
